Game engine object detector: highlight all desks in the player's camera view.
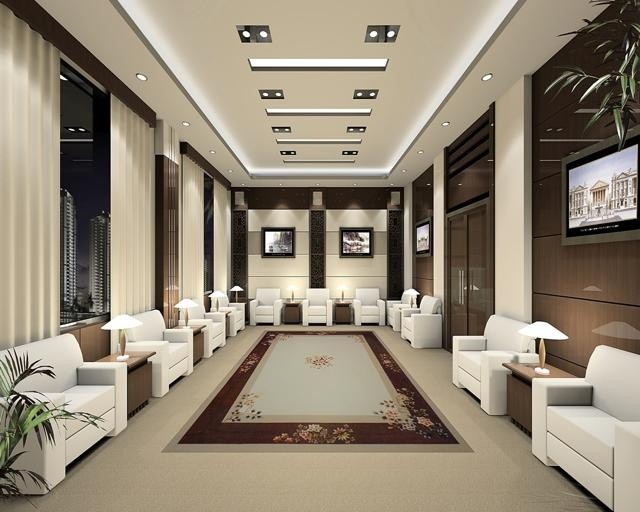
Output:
[95,350,157,421]
[501,361,578,440]
[172,325,207,367]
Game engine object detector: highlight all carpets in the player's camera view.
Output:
[159,329,476,453]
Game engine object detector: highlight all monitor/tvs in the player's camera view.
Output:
[261,227,296,258]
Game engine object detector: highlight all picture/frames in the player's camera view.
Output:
[260,225,296,259]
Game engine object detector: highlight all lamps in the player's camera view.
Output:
[100,313,144,361]
[515,320,570,376]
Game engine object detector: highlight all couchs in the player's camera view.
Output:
[530,343,640,511]
[173,285,443,359]
[452,314,540,416]
[117,309,193,397]
[0,333,128,495]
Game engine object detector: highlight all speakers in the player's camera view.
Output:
[235,192,244,205]
[313,191,322,205]
[391,191,400,205]
